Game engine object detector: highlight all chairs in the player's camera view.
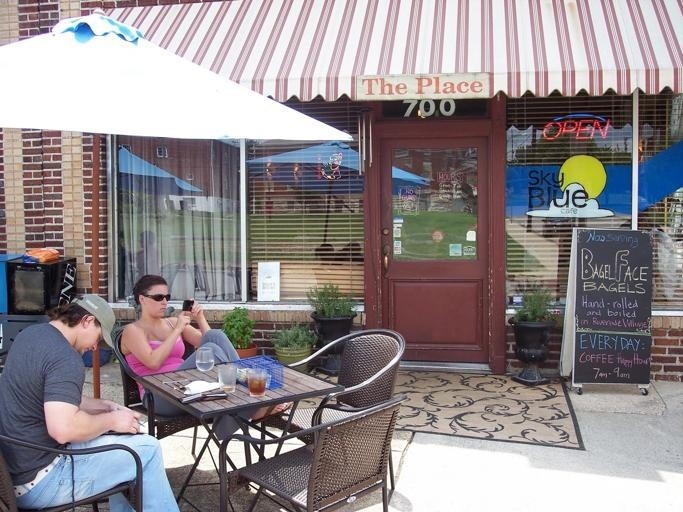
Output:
[113,328,213,454]
[259,328,406,491]
[0,435,142,512]
[219,396,407,512]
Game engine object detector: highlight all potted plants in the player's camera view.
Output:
[508,286,559,387]
[271,325,318,372]
[305,283,357,377]
[221,306,258,359]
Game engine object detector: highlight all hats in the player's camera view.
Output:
[71,294,116,350]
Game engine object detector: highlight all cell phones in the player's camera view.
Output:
[182,300,194,312]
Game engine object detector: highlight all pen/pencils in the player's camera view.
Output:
[117,408,144,427]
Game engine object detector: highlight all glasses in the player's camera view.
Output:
[142,294,171,302]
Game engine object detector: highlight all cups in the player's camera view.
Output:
[195,347,214,371]
[248,368,266,397]
[218,363,236,392]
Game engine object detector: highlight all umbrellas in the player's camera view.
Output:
[0,15,355,397]
[114,141,202,199]
[247,141,430,246]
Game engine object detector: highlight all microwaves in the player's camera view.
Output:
[7,257,77,314]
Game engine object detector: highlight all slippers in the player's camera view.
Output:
[251,402,291,424]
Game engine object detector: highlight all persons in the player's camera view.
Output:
[0,291,183,512]
[312,239,361,265]
[132,228,169,286]
[120,276,291,444]
[118,227,130,282]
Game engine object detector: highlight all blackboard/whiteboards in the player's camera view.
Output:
[572,228,652,388]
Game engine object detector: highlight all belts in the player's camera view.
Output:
[14,454,62,498]
